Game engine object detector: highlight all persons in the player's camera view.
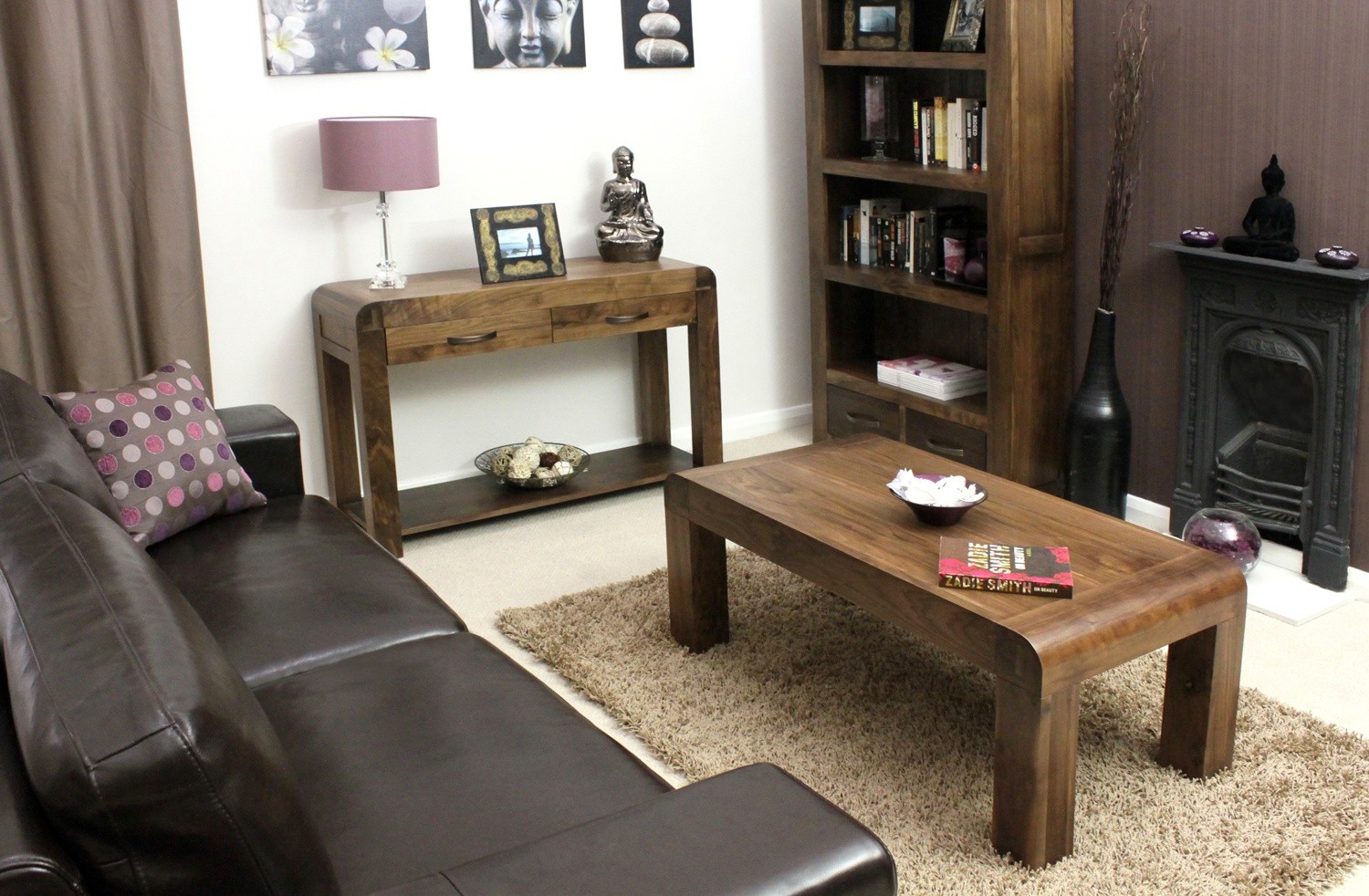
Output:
[526,233,534,256]
[594,146,664,264]
[1223,154,1298,262]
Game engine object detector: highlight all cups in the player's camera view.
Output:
[1182,507,1262,576]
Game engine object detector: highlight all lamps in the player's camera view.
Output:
[317,115,440,291]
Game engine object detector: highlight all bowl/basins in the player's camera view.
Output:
[889,474,987,525]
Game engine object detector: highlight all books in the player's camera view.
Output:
[909,96,987,172]
[937,537,1072,599]
[876,355,988,401]
[836,198,939,288]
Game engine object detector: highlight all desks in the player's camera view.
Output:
[662,429,1249,875]
[310,250,724,560]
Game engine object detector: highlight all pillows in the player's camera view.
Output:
[40,356,268,550]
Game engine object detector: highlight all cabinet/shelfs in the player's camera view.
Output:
[801,0,1081,500]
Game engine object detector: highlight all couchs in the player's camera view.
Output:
[1,366,897,896]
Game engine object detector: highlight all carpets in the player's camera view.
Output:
[495,540,1368,896]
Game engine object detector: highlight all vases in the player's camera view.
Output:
[1064,305,1133,520]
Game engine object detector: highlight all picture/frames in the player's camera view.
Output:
[470,201,567,287]
[842,0,914,52]
[941,0,985,53]
